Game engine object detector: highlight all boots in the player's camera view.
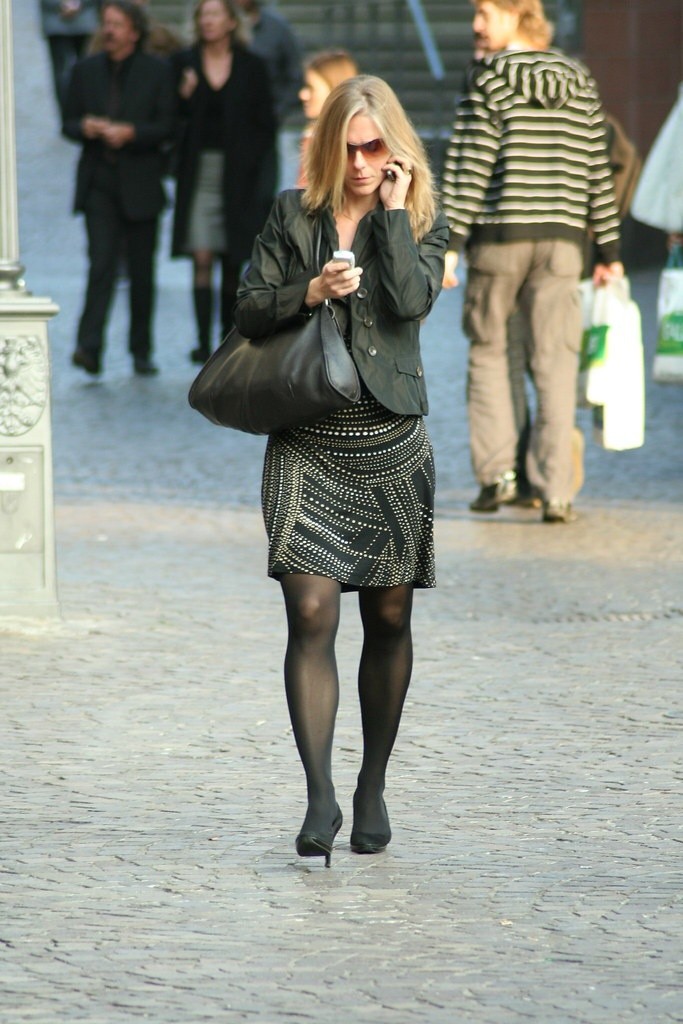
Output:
[188,287,212,362]
[220,290,237,341]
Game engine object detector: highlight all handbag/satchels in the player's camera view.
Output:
[577,274,647,451]
[650,240,683,383]
[188,208,362,436]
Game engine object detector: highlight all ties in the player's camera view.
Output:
[103,63,124,165]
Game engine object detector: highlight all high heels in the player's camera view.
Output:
[295,802,345,868]
[350,790,392,854]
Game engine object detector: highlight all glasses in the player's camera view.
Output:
[345,138,388,163]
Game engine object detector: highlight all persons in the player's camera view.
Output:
[37,0,300,167]
[60,1,178,379]
[159,0,284,366]
[444,1,642,526]
[235,73,450,870]
[628,82,683,254]
[291,49,361,187]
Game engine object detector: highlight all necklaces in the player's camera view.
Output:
[343,214,360,224]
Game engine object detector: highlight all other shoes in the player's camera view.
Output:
[132,355,159,375]
[543,502,579,523]
[469,481,516,509]
[73,348,99,372]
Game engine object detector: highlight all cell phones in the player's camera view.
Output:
[386,161,401,181]
[331,250,355,268]
